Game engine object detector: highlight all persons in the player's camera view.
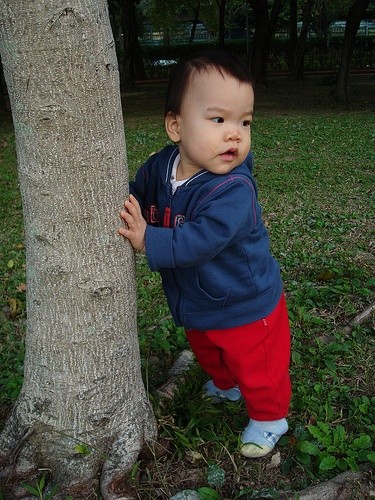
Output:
[119,50,291,459]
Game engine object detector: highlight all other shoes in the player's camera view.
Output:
[238,418,289,457]
[200,379,241,403]
